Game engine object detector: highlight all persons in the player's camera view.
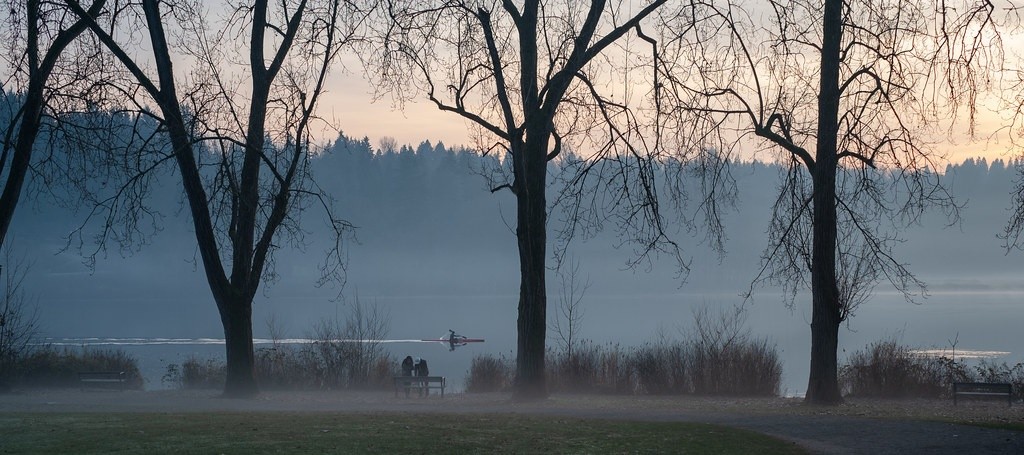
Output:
[449,329,466,340]
[402,355,415,397]
[414,356,429,397]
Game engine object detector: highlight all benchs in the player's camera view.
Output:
[79,371,127,393]
[953,382,1012,408]
[395,375,446,398]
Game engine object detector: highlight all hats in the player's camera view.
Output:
[414,357,420,364]
